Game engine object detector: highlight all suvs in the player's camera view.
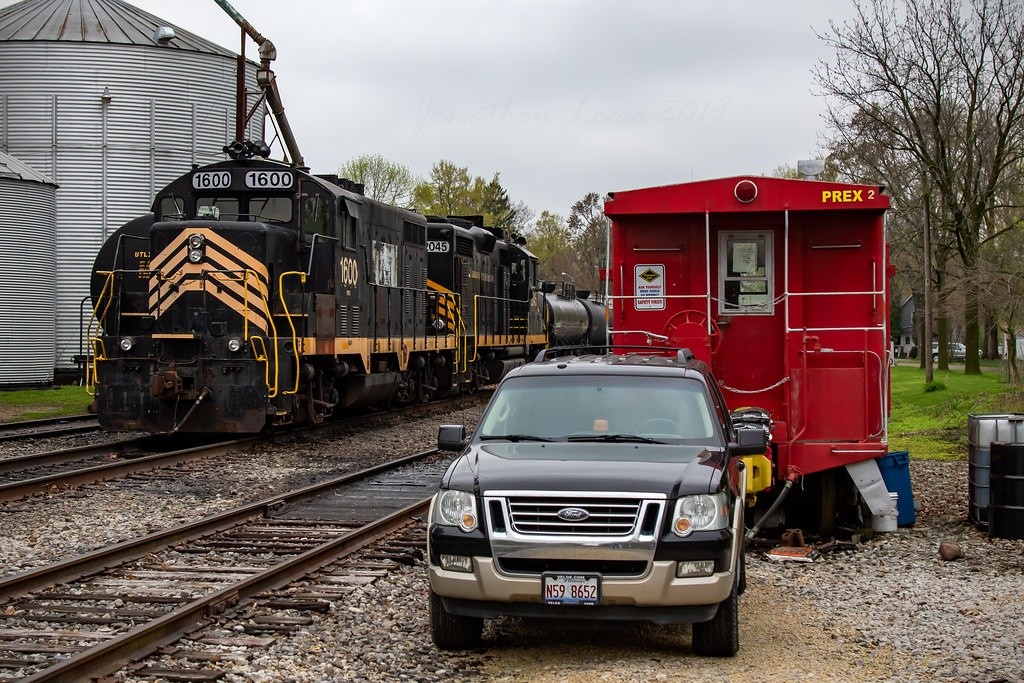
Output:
[426,345,773,658]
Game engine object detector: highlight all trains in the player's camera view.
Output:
[78,1,608,437]
[599,176,896,543]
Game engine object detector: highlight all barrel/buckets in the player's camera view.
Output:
[875,451,916,526]
[989,441,1024,541]
[872,492,899,532]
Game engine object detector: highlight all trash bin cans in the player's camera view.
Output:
[876,451,916,526]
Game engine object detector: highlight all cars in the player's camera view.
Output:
[931,341,983,363]
